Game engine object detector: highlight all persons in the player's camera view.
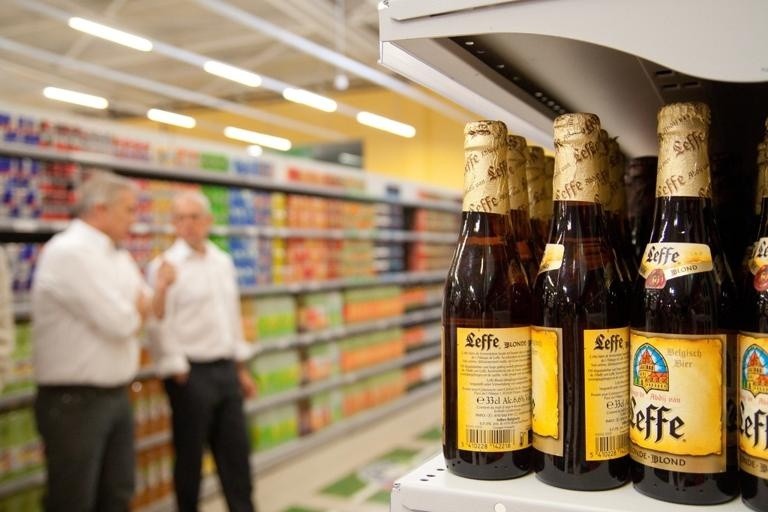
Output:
[32,173,176,510]
[137,189,258,510]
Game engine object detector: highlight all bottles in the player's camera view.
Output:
[439,100,768,512]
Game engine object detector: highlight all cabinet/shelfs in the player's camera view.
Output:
[0,104,462,511]
[377,2,768,510]
